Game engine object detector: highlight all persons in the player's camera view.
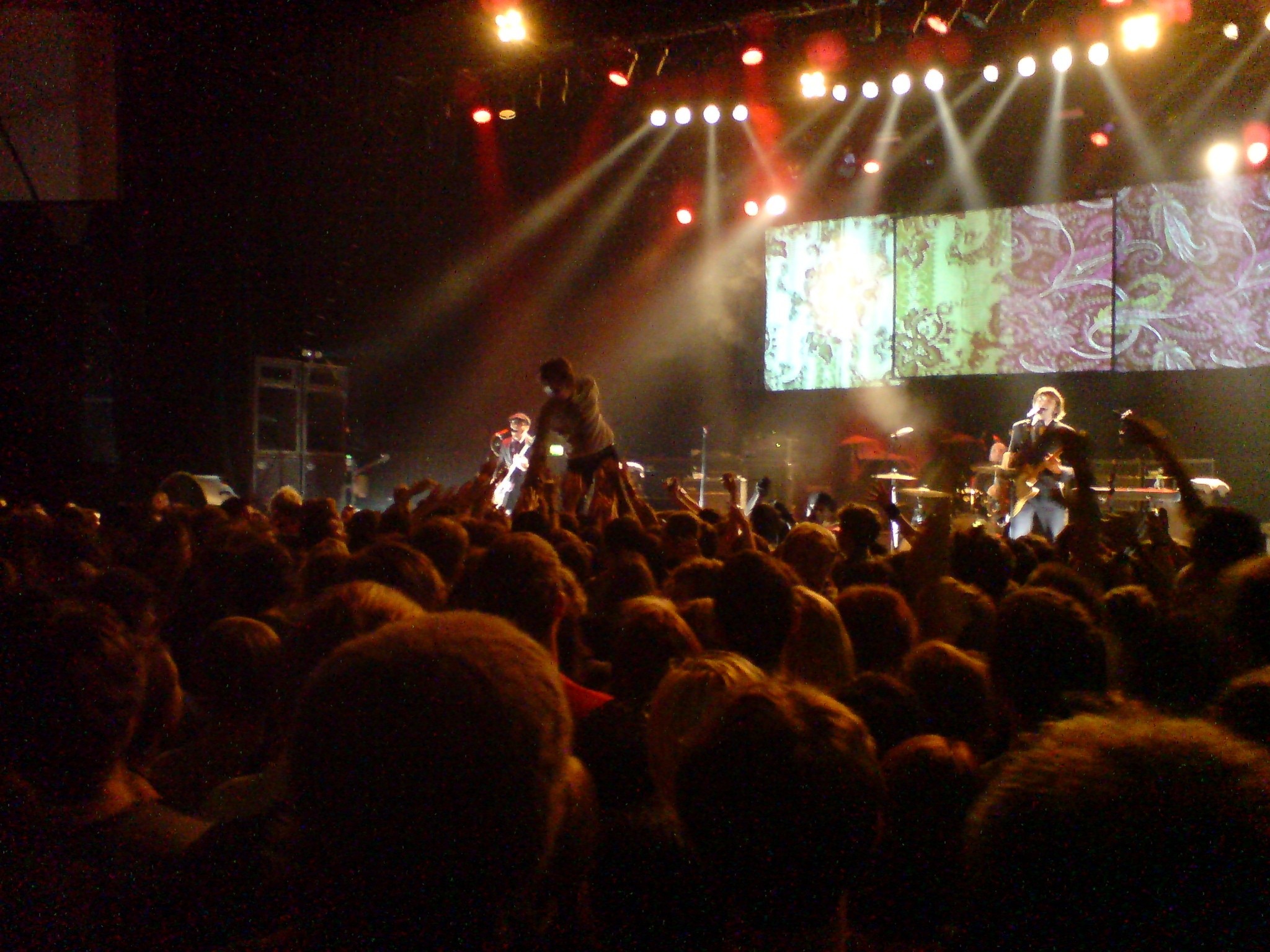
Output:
[995,386,1090,538]
[0,413,1269,952]
[530,360,622,495]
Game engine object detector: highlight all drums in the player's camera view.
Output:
[897,502,954,552]
[956,487,982,514]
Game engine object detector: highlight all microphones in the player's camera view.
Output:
[495,426,512,437]
[1026,406,1046,417]
[889,428,914,438]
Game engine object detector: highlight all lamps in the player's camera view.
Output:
[926,0,1000,33]
[609,44,670,87]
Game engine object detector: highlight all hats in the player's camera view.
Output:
[510,413,530,424]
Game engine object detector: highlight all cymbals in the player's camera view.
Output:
[896,487,953,499]
[970,463,1019,475]
[869,472,919,482]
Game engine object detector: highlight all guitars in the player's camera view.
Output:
[987,446,1063,527]
[491,435,534,509]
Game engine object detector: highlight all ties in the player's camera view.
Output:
[1035,420,1043,440]
